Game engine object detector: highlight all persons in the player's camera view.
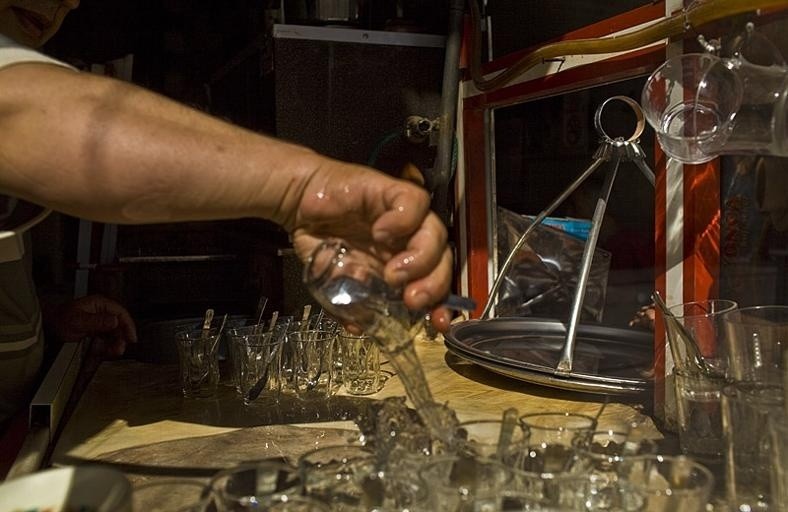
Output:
[0,0,455,453]
[373,112,440,190]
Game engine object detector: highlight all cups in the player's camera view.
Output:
[641,49,788,165]
[302,220,429,359]
[166,307,387,407]
[2,409,712,511]
[660,298,788,511]
